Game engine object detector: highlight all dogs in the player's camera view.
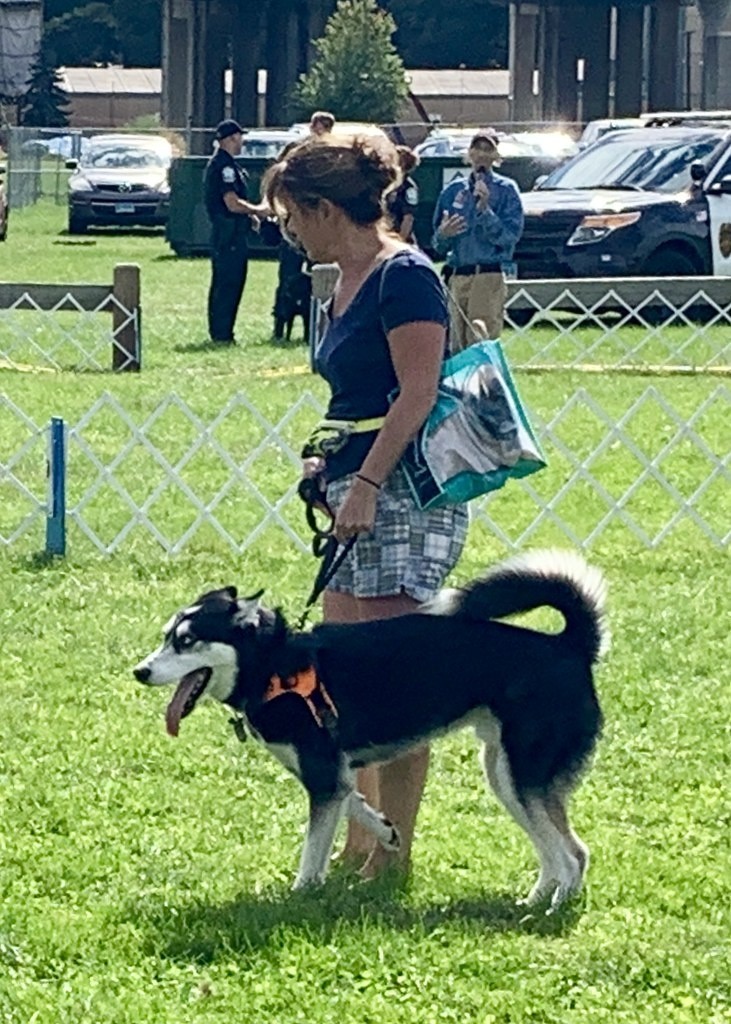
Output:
[131,550,612,915]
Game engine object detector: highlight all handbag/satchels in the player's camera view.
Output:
[379,254,547,510]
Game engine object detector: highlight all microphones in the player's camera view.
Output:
[474,165,486,203]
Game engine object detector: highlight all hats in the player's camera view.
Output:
[216,119,248,142]
[471,129,499,149]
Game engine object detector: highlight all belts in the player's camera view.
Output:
[443,262,502,275]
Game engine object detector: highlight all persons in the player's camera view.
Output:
[260,133,473,893]
[202,109,526,343]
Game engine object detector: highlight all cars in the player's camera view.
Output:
[64,133,181,235]
[237,111,731,329]
[23,135,87,165]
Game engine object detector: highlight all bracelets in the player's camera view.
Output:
[355,472,382,492]
